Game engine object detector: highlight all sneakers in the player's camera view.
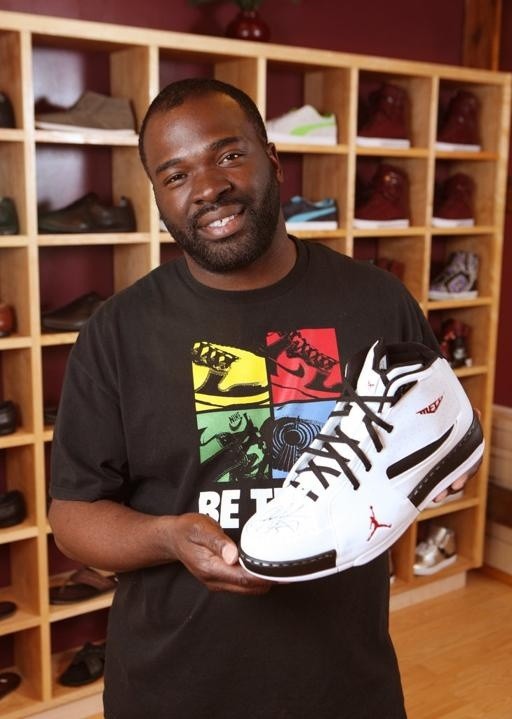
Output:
[0,90,137,338]
[411,525,461,577]
[280,195,338,231]
[435,317,474,371]
[263,103,338,147]
[237,338,485,586]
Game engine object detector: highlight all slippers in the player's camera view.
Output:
[0,400,123,702]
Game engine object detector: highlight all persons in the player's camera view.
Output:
[45,76,477,719]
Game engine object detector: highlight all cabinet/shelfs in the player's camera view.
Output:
[0,11,512,719]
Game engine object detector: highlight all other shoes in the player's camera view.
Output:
[352,162,412,230]
[364,255,407,285]
[428,247,481,300]
[431,172,477,229]
[435,89,481,153]
[355,84,412,149]
